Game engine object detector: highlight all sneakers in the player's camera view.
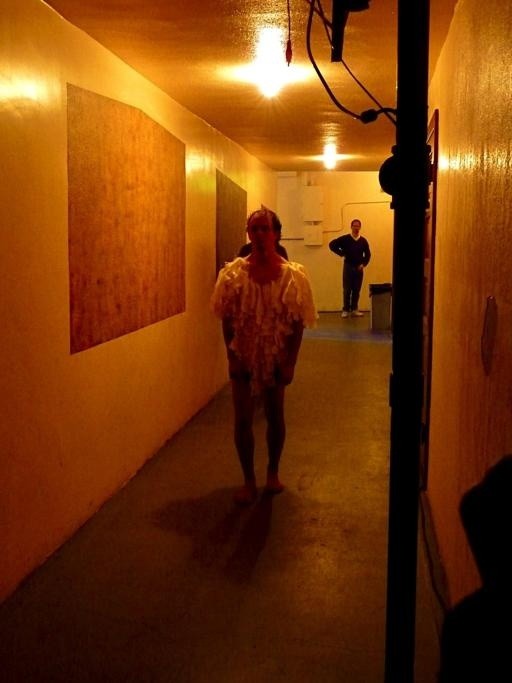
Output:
[341,310,364,318]
[234,471,285,497]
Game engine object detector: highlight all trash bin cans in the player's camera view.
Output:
[369,284,392,331]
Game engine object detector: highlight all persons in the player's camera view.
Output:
[436,454,512,683]
[329,219,370,317]
[210,207,319,494]
